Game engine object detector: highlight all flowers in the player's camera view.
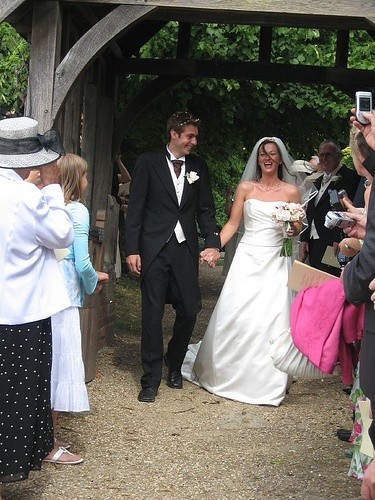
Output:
[272,202,305,258]
[184,171,200,184]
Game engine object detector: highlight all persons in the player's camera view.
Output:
[41,153,109,464]
[124,110,221,403]
[339,108,375,500]
[0,116,74,483]
[199,136,318,407]
[290,141,362,278]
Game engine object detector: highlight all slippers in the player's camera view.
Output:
[41,446,84,464]
[53,436,70,449]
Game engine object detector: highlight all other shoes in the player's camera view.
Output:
[338,428,352,442]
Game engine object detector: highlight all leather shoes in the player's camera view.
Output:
[137,387,158,402]
[168,362,183,389]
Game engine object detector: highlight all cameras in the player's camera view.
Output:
[355,91,372,125]
[338,190,347,199]
[324,211,355,230]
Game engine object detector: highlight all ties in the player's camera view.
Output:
[321,175,331,192]
[164,147,183,179]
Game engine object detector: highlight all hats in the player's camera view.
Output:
[0,117,66,169]
